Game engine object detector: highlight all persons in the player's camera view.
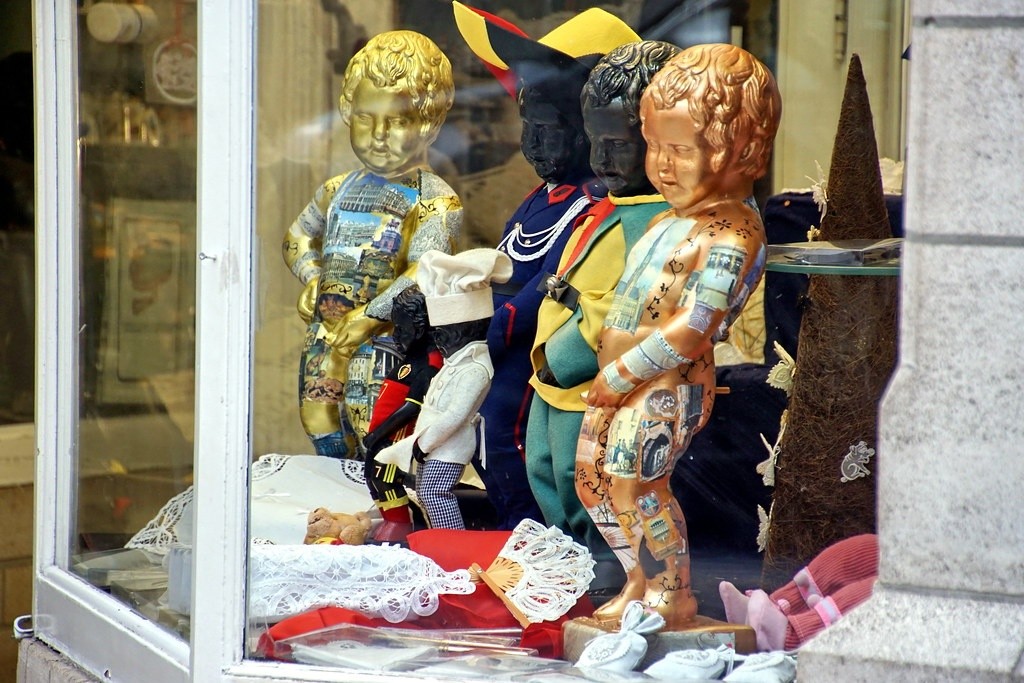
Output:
[282,2,877,652]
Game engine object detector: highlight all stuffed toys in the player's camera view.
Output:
[304,507,371,545]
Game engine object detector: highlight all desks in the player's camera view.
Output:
[762,238,900,277]
